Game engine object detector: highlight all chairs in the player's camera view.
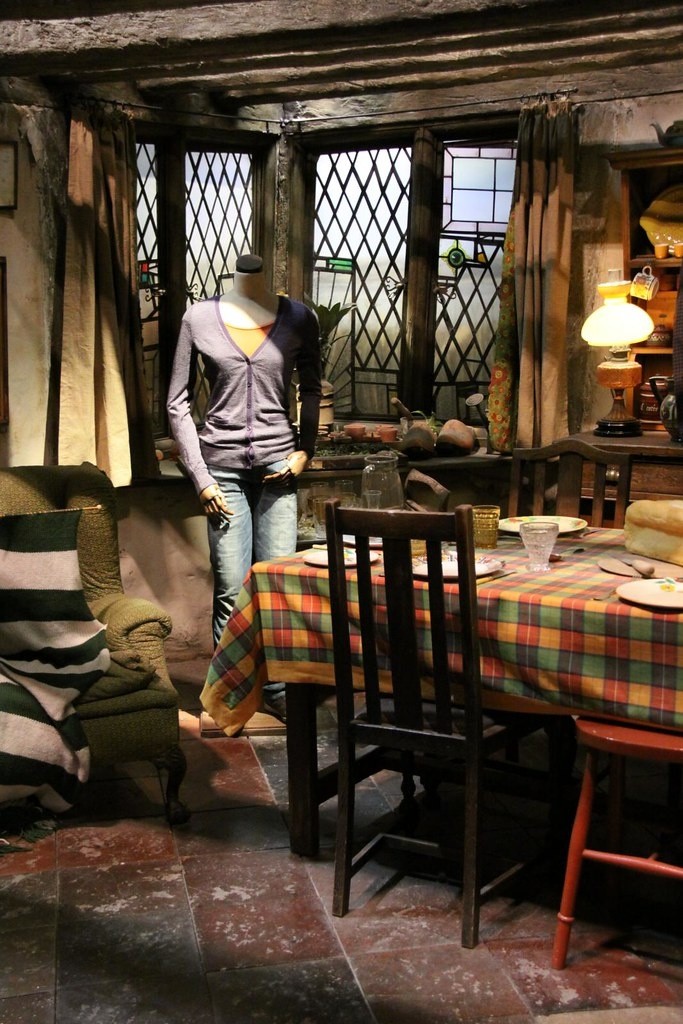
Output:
[508,437,634,530]
[322,497,577,949]
[0,459,192,854]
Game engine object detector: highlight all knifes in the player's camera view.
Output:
[607,551,654,576]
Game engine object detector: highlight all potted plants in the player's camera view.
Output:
[297,291,357,427]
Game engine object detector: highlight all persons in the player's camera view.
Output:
[168,254,323,736]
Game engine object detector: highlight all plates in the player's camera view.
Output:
[342,534,383,548]
[615,579,683,609]
[303,548,379,567]
[497,515,588,534]
[412,550,503,579]
[597,559,683,579]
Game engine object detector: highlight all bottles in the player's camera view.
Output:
[361,454,405,510]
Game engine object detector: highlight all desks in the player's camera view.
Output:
[158,444,511,487]
[199,526,683,857]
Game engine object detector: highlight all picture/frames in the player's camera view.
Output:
[0,140,18,210]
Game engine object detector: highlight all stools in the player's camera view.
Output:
[552,715,683,972]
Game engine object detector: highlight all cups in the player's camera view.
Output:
[472,505,500,550]
[629,265,659,301]
[654,243,669,259]
[298,480,382,539]
[674,243,683,258]
[519,521,559,572]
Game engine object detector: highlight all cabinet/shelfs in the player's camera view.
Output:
[552,146,683,528]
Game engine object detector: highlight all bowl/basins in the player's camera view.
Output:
[643,324,673,348]
[638,373,668,421]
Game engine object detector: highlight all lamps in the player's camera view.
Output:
[580,270,653,437]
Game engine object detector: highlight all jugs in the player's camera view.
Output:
[465,394,501,455]
